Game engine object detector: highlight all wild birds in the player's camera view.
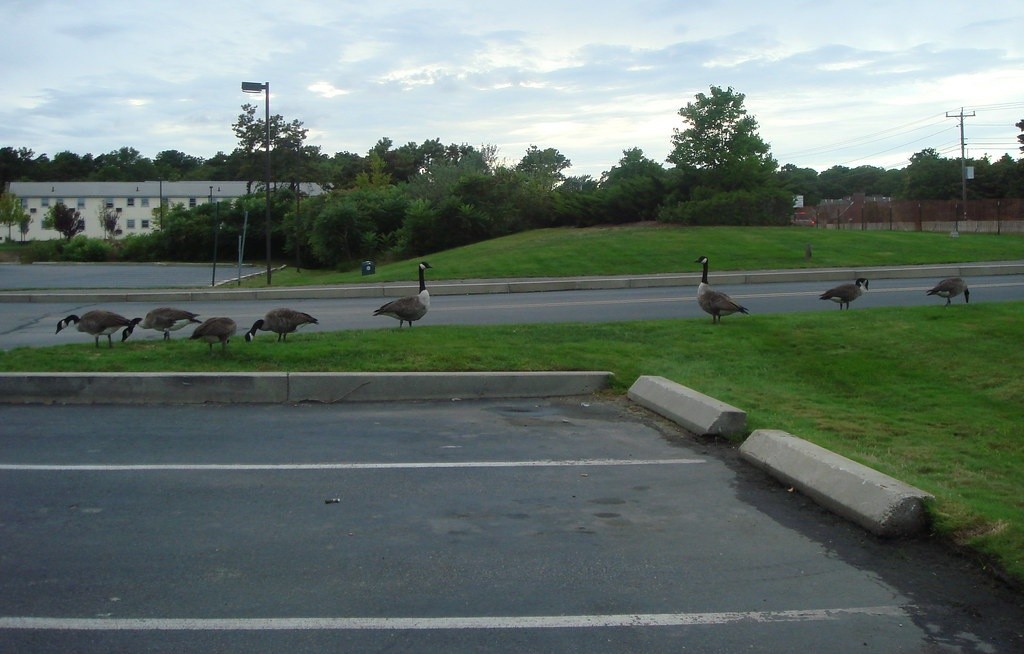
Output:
[55,307,318,356]
[819,278,870,309]
[926,277,969,305]
[370,262,433,326]
[695,256,752,322]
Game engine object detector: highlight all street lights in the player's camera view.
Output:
[241,80,272,286]
